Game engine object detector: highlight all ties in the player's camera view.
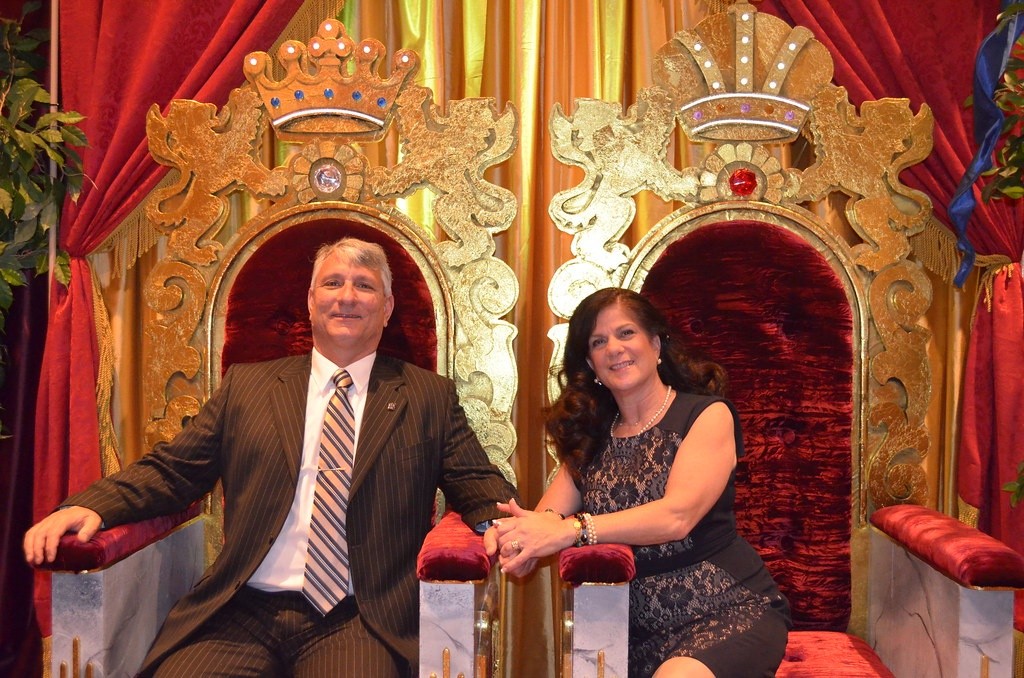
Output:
[302,369,354,617]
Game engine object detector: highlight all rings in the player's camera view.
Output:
[511,540,520,551]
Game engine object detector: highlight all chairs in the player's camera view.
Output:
[33,17,522,678]
[546,0,1023,678]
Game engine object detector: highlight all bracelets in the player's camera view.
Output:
[574,512,597,547]
[545,509,565,520]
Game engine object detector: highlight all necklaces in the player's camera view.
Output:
[610,385,671,437]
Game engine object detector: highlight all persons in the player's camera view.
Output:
[492,287,793,677]
[24,240,540,678]
